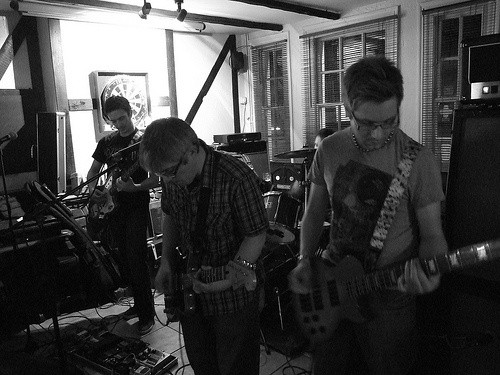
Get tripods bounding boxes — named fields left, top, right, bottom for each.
left=0, top=150, right=57, bottom=355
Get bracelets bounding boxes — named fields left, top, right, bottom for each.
left=298, top=254, right=311, bottom=263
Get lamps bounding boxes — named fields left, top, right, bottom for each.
left=175, top=0, right=187, bottom=21
left=138, top=0, right=151, bottom=19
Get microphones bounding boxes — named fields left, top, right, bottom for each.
left=0, top=131, right=18, bottom=144
left=467, top=332, right=493, bottom=344
left=110, top=142, right=141, bottom=158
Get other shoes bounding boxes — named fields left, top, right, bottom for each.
left=136, top=315, right=153, bottom=334
left=124, top=305, right=137, bottom=316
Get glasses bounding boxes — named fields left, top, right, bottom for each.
left=153, top=156, right=183, bottom=178
left=350, top=108, right=400, bottom=131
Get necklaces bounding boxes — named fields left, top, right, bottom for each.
left=352, top=131, right=394, bottom=152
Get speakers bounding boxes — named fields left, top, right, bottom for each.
left=231, top=52, right=244, bottom=69
left=443, top=104, right=500, bottom=375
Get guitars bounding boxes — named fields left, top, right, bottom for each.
left=164, top=255, right=257, bottom=323
left=88, top=157, right=144, bottom=220
left=292, top=240, right=499, bottom=345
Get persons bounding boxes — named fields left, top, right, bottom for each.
left=87, top=96, right=156, bottom=334
left=289, top=55, right=447, bottom=375
left=291, top=128, right=336, bottom=222
left=139, top=118, right=271, bottom=375
